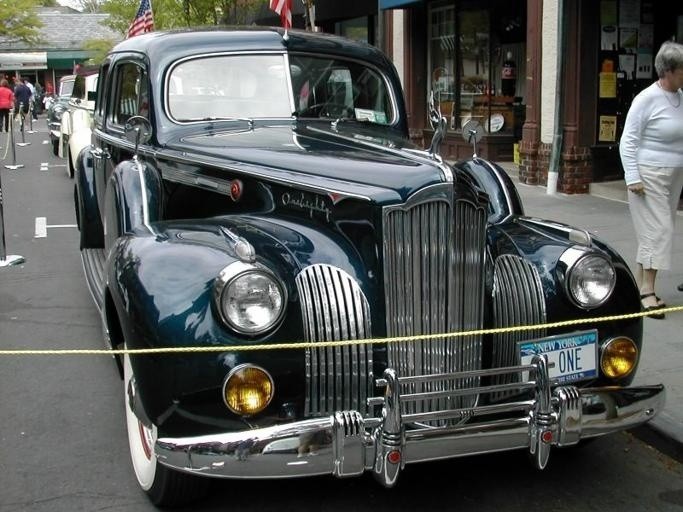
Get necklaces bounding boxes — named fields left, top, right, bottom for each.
left=658, top=80, right=681, bottom=108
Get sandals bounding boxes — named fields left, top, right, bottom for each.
left=640, top=292, right=665, bottom=319
left=657, top=297, right=667, bottom=310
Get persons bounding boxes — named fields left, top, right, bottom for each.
left=0, top=79, right=14, bottom=133
left=13, top=78, right=32, bottom=132
left=19, top=77, right=38, bottom=127
left=619, top=41, right=683, bottom=320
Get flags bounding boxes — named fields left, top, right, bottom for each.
left=126, top=0, right=155, bottom=38
left=270, top=0, right=293, bottom=27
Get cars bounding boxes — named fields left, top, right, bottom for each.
left=58, top=65, right=98, bottom=178
left=73, top=26, right=665, bottom=508
left=47, top=75, right=76, bottom=154
